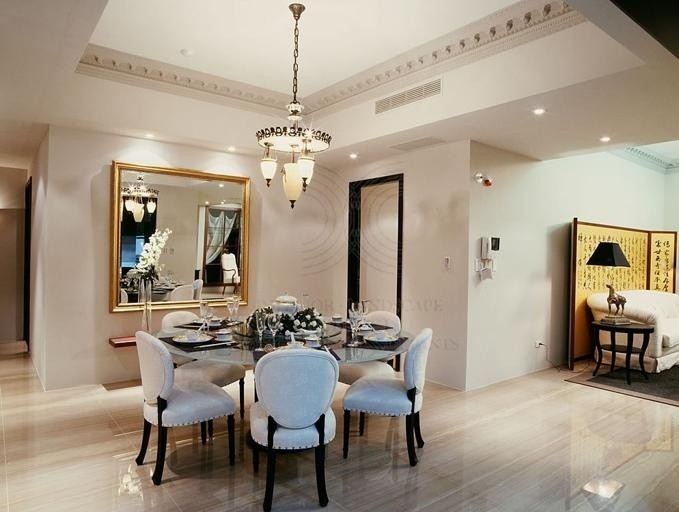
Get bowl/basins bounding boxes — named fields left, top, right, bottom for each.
left=304, top=337, right=321, bottom=348
left=331, top=315, right=343, bottom=325
left=186, top=330, right=200, bottom=341
left=374, top=330, right=386, bottom=340
left=215, top=329, right=233, bottom=341
left=211, top=318, right=222, bottom=326
left=287, top=341, right=304, bottom=348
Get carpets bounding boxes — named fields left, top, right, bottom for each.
left=562, top=363, right=679, bottom=407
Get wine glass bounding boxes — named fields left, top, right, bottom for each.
left=225, top=295, right=240, bottom=326
left=254, top=310, right=266, bottom=352
left=267, top=313, right=281, bottom=350
left=199, top=301, right=214, bottom=335
left=346, top=299, right=373, bottom=348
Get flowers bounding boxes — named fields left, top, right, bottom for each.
left=124, top=227, right=174, bottom=330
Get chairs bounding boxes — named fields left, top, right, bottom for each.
left=218, top=250, right=241, bottom=295
left=164, top=278, right=203, bottom=303
left=587, top=287, right=678, bottom=375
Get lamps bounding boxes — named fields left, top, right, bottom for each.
left=254, top=3, right=332, bottom=213
left=120, top=170, right=161, bottom=223
left=474, top=170, right=495, bottom=187
left=585, top=241, right=634, bottom=325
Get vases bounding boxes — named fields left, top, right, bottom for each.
left=139, top=276, right=153, bottom=334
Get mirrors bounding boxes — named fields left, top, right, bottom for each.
left=107, top=159, right=252, bottom=313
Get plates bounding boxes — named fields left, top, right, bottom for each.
left=363, top=335, right=399, bottom=343
left=171, top=334, right=214, bottom=344
left=192, top=317, right=223, bottom=324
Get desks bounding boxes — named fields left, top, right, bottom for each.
left=589, top=319, right=656, bottom=385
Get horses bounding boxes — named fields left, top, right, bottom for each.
left=605, top=282, right=627, bottom=317
